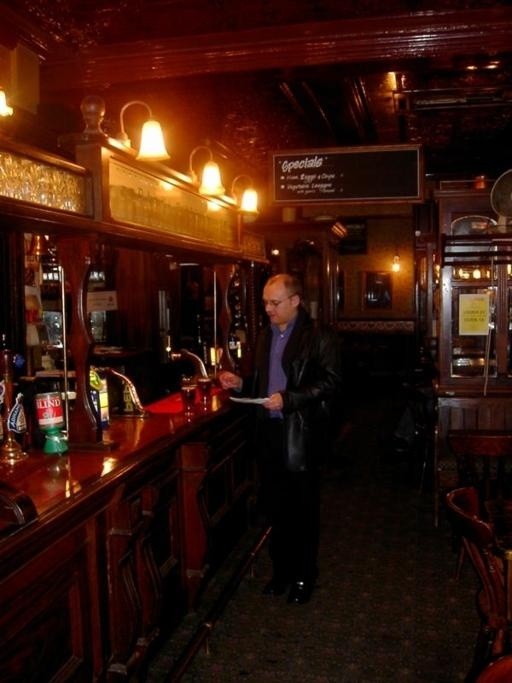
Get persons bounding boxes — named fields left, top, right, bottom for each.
left=218, top=272, right=342, bottom=606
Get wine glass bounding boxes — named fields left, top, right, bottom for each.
left=181, top=378, right=213, bottom=423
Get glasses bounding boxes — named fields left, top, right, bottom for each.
left=263, top=291, right=297, bottom=307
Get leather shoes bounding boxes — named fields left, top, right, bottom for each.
left=261, top=575, right=316, bottom=606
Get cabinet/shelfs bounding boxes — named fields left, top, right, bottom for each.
left=416, top=180, right=512, bottom=526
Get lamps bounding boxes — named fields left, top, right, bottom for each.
left=232, top=175, right=260, bottom=215
left=0, top=90, right=13, bottom=116
left=116, top=100, right=171, bottom=163
left=184, top=145, right=226, bottom=197
left=392, top=253, right=400, bottom=272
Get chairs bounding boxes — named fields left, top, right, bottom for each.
left=445, top=487, right=512, bottom=675
